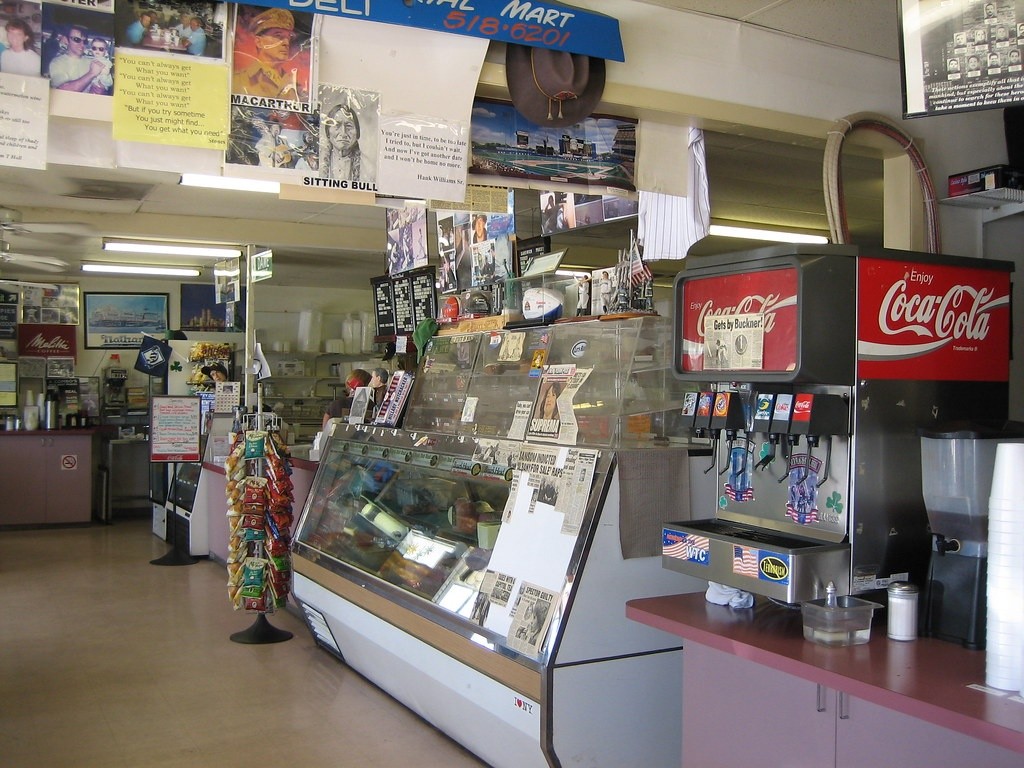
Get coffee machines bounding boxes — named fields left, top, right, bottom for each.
left=102, top=366, right=129, bottom=416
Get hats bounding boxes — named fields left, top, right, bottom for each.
left=472, top=214, right=487, bottom=230
left=201, top=363, right=227, bottom=380
left=505, top=43, right=606, bottom=128
left=412, top=316, right=438, bottom=364
left=249, top=7, right=298, bottom=35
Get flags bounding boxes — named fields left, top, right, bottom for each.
left=631, top=233, right=653, bottom=289
left=133, top=335, right=173, bottom=377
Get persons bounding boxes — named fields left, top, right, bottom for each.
left=201, top=363, right=227, bottom=392
left=599, top=271, right=612, bottom=315
left=0, top=18, right=41, bottom=77
left=948, top=3, right=1024, bottom=74
left=529, top=382, right=560, bottom=435
left=44, top=25, right=114, bottom=94
left=573, top=273, right=589, bottom=317
left=255, top=120, right=296, bottom=168
left=544, top=195, right=560, bottom=234
left=126, top=10, right=206, bottom=56
left=318, top=106, right=374, bottom=183
left=442, top=254, right=456, bottom=291
left=233, top=8, right=302, bottom=101
left=372, top=368, right=388, bottom=410
left=322, top=369, right=376, bottom=431
left=472, top=214, right=487, bottom=243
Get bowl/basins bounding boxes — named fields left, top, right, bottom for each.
left=800, top=595, right=884, bottom=648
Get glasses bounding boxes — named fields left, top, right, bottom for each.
left=91, top=46, right=104, bottom=51
left=69, top=36, right=88, bottom=45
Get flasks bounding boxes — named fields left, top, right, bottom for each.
left=44, top=389, right=57, bottom=430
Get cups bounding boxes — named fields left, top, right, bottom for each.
left=477, top=521, right=501, bottom=549
left=293, top=422, right=300, bottom=439
left=26, top=390, right=33, bottom=405
left=986, top=443, right=1024, bottom=692
left=282, top=429, right=288, bottom=446
left=37, top=394, right=44, bottom=422
left=288, top=433, right=295, bottom=444
left=331, top=364, right=338, bottom=377
left=124, top=436, right=129, bottom=440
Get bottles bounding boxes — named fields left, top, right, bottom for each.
left=6, top=416, right=14, bottom=431
left=24, top=412, right=39, bottom=432
left=232, top=410, right=244, bottom=448
left=58, top=413, right=62, bottom=430
left=111, top=354, right=120, bottom=369
left=886, top=581, right=920, bottom=641
left=80, top=410, right=88, bottom=429
left=15, top=416, right=22, bottom=430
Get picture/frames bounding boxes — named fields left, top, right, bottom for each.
left=83, top=291, right=169, bottom=349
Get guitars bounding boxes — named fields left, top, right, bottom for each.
left=258, top=144, right=306, bottom=169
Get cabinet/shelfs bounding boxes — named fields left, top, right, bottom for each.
left=0, top=434, right=95, bottom=532
left=682, top=637, right=1024, bottom=768
